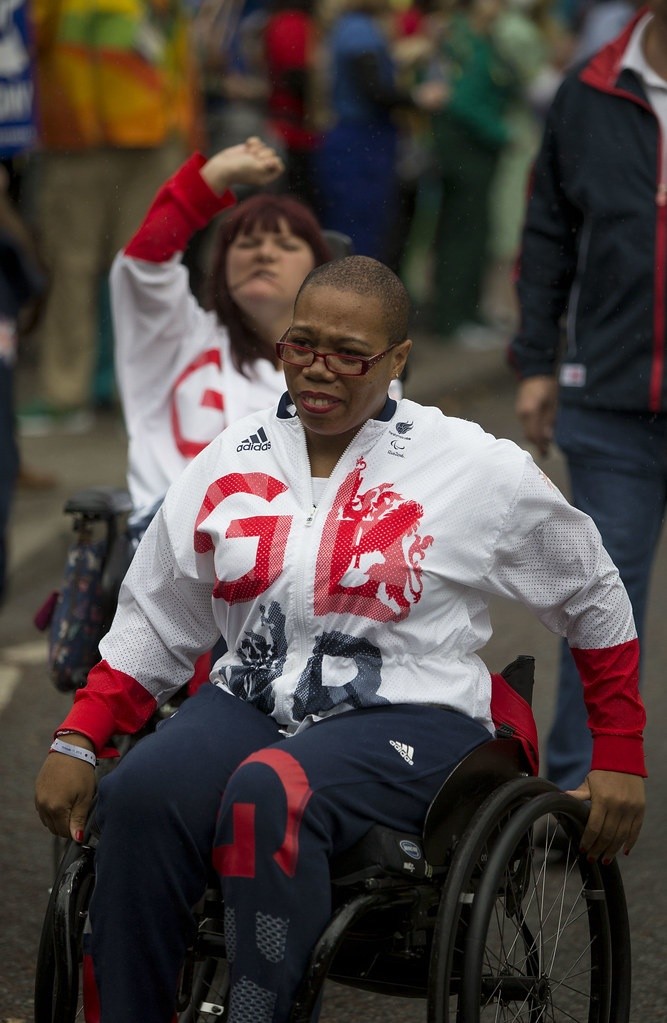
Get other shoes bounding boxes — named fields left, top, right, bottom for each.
left=534, top=812, right=577, bottom=850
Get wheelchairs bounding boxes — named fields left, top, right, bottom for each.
left=32, top=654, right=632, bottom=1023
left=47, top=488, right=136, bottom=692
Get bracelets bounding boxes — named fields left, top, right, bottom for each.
left=49, top=738, right=96, bottom=767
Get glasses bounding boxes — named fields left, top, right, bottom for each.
left=276, top=325, right=401, bottom=376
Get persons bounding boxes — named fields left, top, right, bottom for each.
left=1, top=0, right=638, bottom=609
left=109, top=136, right=329, bottom=715
left=504, top=0, right=667, bottom=867
left=32, top=255, right=648, bottom=1023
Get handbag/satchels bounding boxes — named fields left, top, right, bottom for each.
left=46, top=537, right=128, bottom=693
